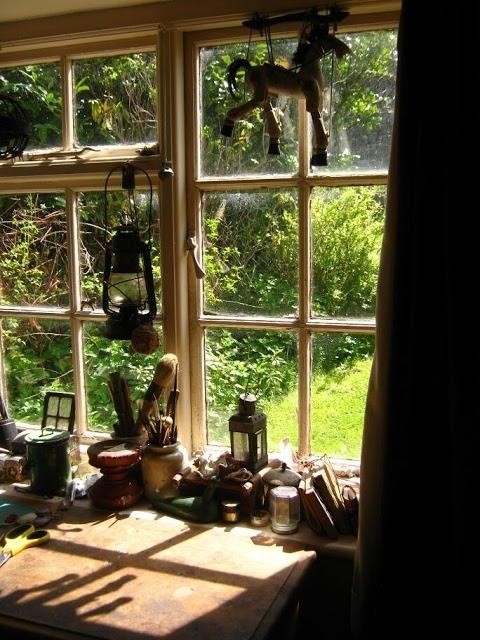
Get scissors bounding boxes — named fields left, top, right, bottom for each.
left=0, top=523, right=50, bottom=568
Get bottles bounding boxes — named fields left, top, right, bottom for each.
left=221, top=499, right=240, bottom=524
left=269, top=486, right=301, bottom=536
left=24, top=428, right=71, bottom=492
left=142, top=441, right=182, bottom=499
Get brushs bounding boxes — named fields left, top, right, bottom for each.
left=130, top=352, right=178, bottom=437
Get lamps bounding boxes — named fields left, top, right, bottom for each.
left=103, top=163, right=156, bottom=340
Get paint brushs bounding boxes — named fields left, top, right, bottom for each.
left=136, top=389, right=180, bottom=448
left=107, top=372, right=135, bottom=437
